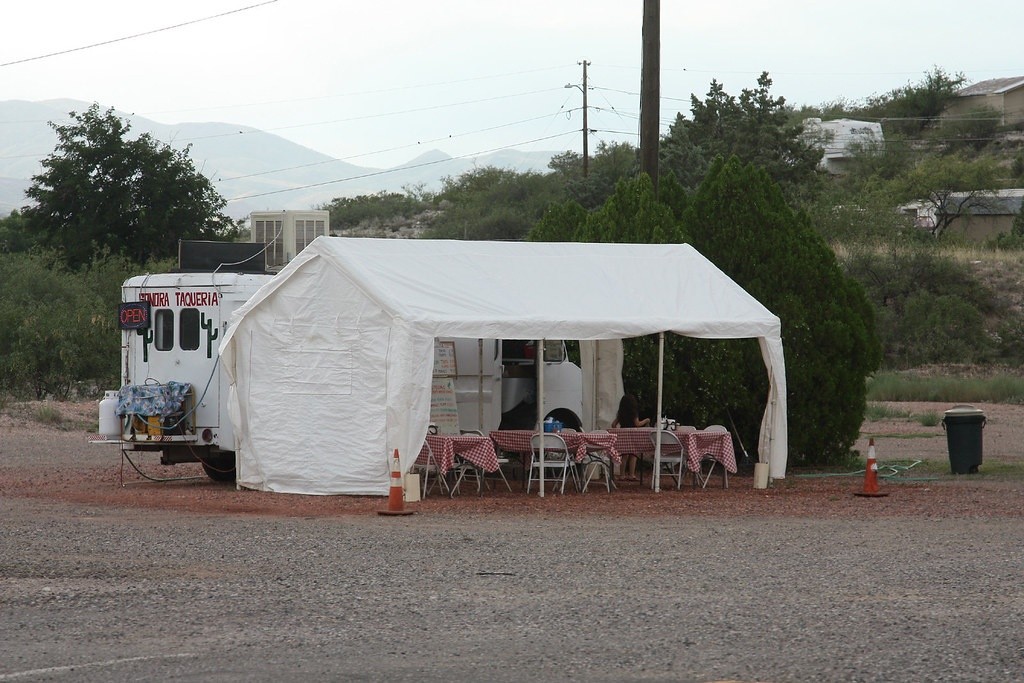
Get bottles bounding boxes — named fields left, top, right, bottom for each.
left=553, top=424, right=560, bottom=433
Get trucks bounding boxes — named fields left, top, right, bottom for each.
left=89, top=208, right=582, bottom=481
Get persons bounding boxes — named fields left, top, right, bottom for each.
left=612, top=394, right=650, bottom=481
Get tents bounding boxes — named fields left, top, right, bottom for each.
left=216, top=235, right=788, bottom=497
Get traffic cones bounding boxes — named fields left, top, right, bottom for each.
left=377, top=448, right=414, bottom=517
left=853, top=438, right=890, bottom=497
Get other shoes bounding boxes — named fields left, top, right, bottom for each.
left=629, top=477, right=641, bottom=482
left=619, top=477, right=629, bottom=481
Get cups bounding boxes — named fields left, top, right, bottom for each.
left=661, top=419, right=677, bottom=431
